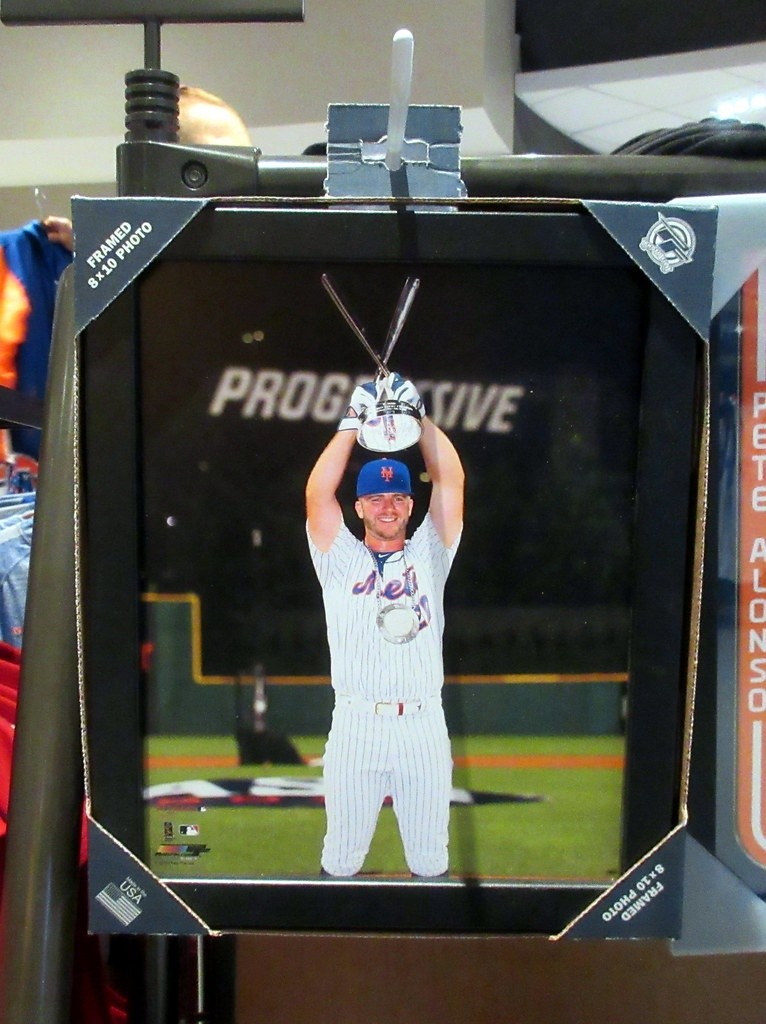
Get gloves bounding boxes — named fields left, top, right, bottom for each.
left=336, top=375, right=384, bottom=430
left=384, top=373, right=426, bottom=419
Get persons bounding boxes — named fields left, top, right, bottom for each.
left=305, top=376, right=466, bottom=880
left=45, top=214, right=73, bottom=255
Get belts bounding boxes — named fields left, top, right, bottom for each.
left=338, top=695, right=442, bottom=717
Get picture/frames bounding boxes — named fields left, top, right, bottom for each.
left=69, top=198, right=717, bottom=940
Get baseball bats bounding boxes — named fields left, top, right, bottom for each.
left=372, top=274, right=423, bottom=384
left=319, top=271, right=391, bottom=377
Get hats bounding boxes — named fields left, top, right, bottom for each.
left=354, top=457, right=415, bottom=498
